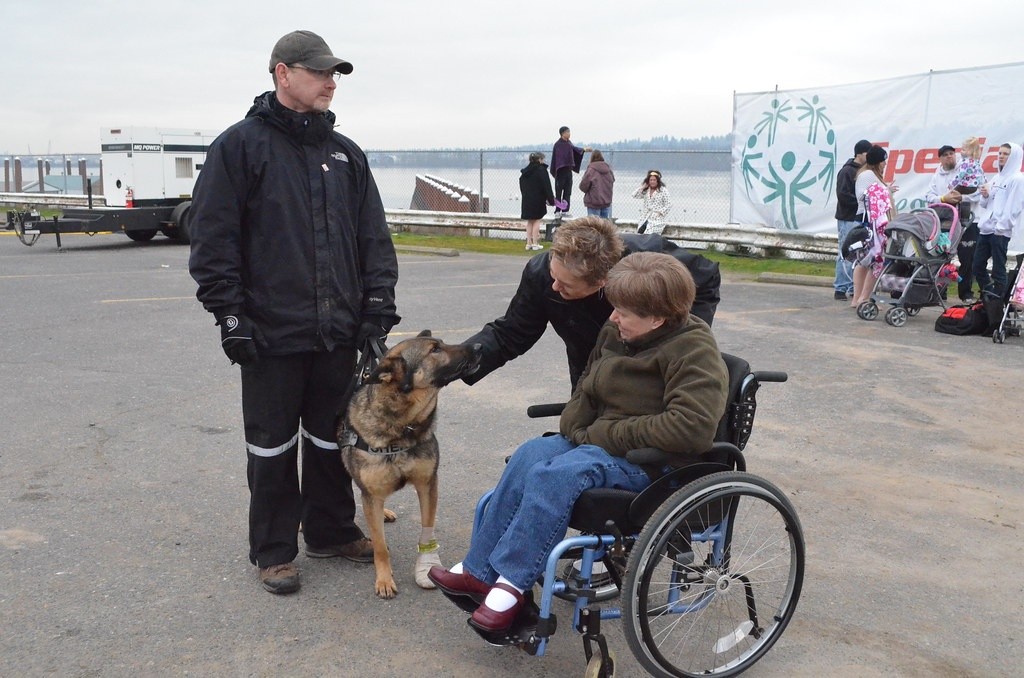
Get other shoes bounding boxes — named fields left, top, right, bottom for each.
left=561, top=211, right=573, bottom=218
left=525, top=244, right=532, bottom=250
left=532, top=243, right=543, bottom=251
left=834, top=290, right=848, bottom=301
left=963, top=299, right=973, bottom=306
left=555, top=211, right=560, bottom=218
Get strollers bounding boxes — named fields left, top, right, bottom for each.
left=992, top=254, right=1024, bottom=344
left=856, top=201, right=976, bottom=328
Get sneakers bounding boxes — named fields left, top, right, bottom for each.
left=304, top=537, right=374, bottom=562
left=256, top=562, right=299, bottom=593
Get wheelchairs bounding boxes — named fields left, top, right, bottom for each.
left=441, top=350, right=806, bottom=678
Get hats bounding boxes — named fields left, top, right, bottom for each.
left=938, top=146, right=955, bottom=157
left=866, top=145, right=888, bottom=164
left=559, top=127, right=569, bottom=134
left=268, top=29, right=353, bottom=75
left=854, top=140, right=872, bottom=154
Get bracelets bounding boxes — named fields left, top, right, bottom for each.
left=941, top=195, right=945, bottom=202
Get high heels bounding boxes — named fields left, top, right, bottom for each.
left=427, top=565, right=492, bottom=605
left=469, top=582, right=527, bottom=634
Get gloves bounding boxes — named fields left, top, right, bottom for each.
left=220, top=305, right=270, bottom=366
left=355, top=322, right=387, bottom=358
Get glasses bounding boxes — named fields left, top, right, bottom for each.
left=285, top=65, right=341, bottom=83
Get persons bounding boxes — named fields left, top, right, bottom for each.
left=851, top=145, right=899, bottom=311
left=189, top=30, right=401, bottom=594
left=631, top=170, right=672, bottom=236
left=927, top=137, right=988, bottom=303
left=579, top=149, right=615, bottom=218
left=550, top=126, right=592, bottom=219
left=833, top=139, right=872, bottom=300
left=459, top=214, right=721, bottom=580
left=428, top=252, right=729, bottom=628
left=971, top=142, right=1024, bottom=300
left=519, top=152, right=554, bottom=251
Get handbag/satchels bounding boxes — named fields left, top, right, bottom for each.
left=546, top=219, right=561, bottom=241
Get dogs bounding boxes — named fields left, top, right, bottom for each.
left=335, top=328, right=484, bottom=602
